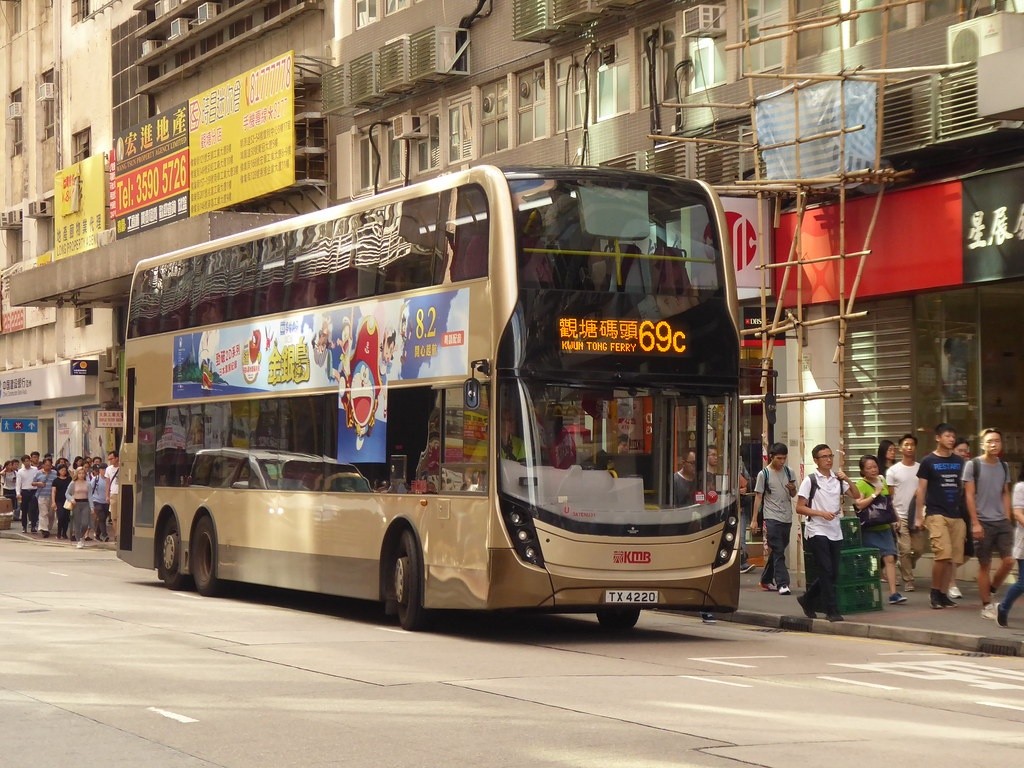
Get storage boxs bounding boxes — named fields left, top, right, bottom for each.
left=801, top=517, right=883, bottom=615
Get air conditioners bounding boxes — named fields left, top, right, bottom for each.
left=28, top=201, right=52, bottom=216
left=417, top=30, right=456, bottom=74
left=171, top=18, right=189, bottom=37
left=384, top=33, right=421, bottom=85
left=683, top=4, right=727, bottom=38
left=520, top=0, right=642, bottom=40
left=393, top=116, right=429, bottom=139
left=142, top=40, right=157, bottom=56
left=354, top=68, right=381, bottom=97
left=0, top=209, right=21, bottom=229
left=947, top=11, right=1024, bottom=65
left=39, top=83, right=54, bottom=99
left=9, top=102, right=21, bottom=118
left=198, top=2, right=217, bottom=25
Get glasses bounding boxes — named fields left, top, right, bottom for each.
left=708, top=453, right=718, bottom=458
left=817, top=454, right=835, bottom=460
left=684, top=460, right=696, bottom=468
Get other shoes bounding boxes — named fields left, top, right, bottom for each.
left=700, top=612, right=716, bottom=623
left=896, top=580, right=901, bottom=586
left=797, top=596, right=818, bottom=618
left=881, top=576, right=887, bottom=582
left=939, top=594, right=958, bottom=607
left=824, top=609, right=843, bottom=621
left=22, top=526, right=110, bottom=549
left=930, top=598, right=944, bottom=608
left=904, top=580, right=915, bottom=592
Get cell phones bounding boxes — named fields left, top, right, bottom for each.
left=789, top=480, right=796, bottom=484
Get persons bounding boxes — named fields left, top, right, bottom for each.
left=795, top=444, right=860, bottom=621
left=915, top=423, right=971, bottom=609
left=0, top=451, right=119, bottom=548
left=672, top=445, right=756, bottom=574
left=750, top=443, right=798, bottom=595
left=878, top=434, right=926, bottom=592
left=854, top=455, right=906, bottom=604
left=961, top=428, right=1016, bottom=621
left=618, top=434, right=631, bottom=455
left=466, top=409, right=527, bottom=492
left=545, top=192, right=594, bottom=292
left=996, top=464, right=1024, bottom=627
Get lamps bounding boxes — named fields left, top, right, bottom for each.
left=55, top=295, right=65, bottom=309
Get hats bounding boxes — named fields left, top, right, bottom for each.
left=93, top=464, right=100, bottom=470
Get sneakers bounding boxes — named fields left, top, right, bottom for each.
left=981, top=604, right=995, bottom=619
left=778, top=585, right=791, bottom=594
left=994, top=602, right=1008, bottom=627
left=948, top=586, right=962, bottom=598
left=888, top=593, right=907, bottom=603
left=759, top=580, right=778, bottom=591
left=740, top=563, right=756, bottom=573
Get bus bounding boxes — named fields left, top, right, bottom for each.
left=116, top=162, right=781, bottom=633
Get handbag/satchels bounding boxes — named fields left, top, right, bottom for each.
left=63, top=498, right=73, bottom=511
left=853, top=480, right=897, bottom=528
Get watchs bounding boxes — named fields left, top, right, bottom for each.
left=871, top=494, right=877, bottom=498
